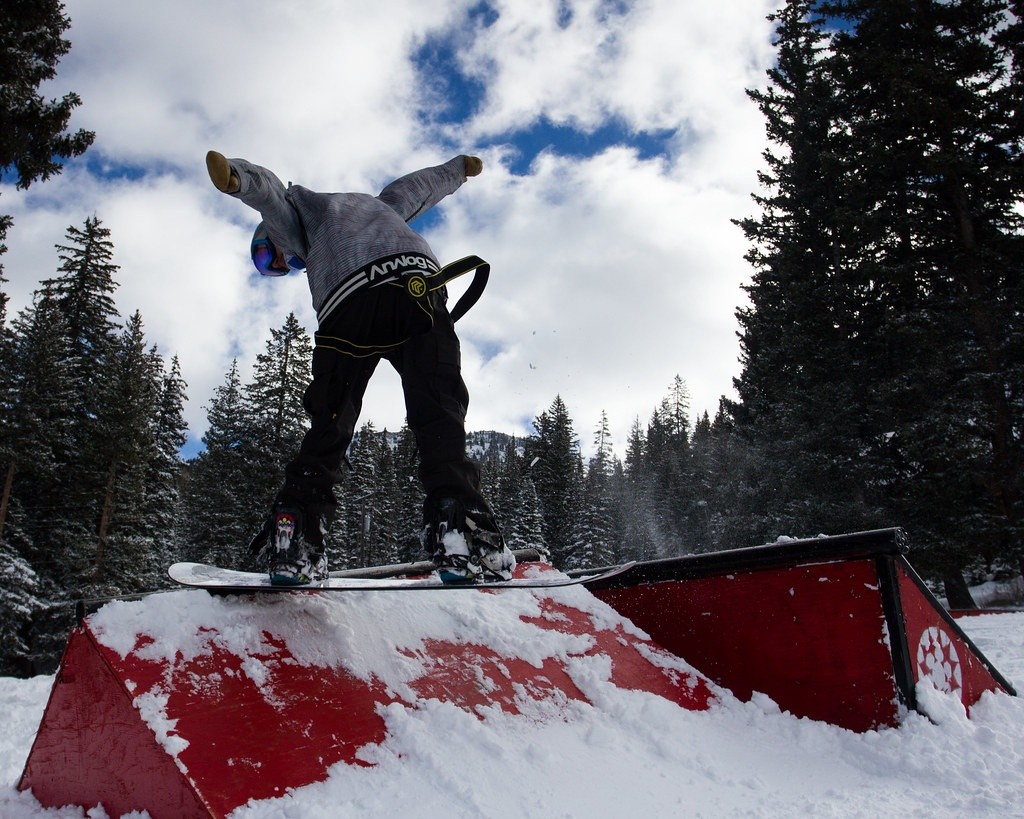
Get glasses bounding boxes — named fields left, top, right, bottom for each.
left=252, top=240, right=287, bottom=276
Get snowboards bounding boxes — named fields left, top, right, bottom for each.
left=167, top=560, right=637, bottom=590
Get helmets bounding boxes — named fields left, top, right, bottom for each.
left=251, top=219, right=301, bottom=278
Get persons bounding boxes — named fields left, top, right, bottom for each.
left=206, top=149, right=515, bottom=586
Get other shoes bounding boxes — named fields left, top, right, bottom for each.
left=439, top=551, right=516, bottom=583
left=270, top=515, right=328, bottom=584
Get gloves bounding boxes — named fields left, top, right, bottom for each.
left=462, top=154, right=483, bottom=177
left=206, top=150, right=240, bottom=193
